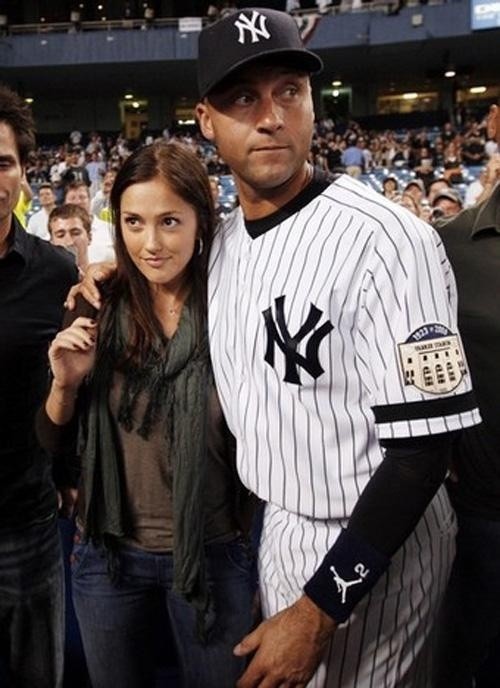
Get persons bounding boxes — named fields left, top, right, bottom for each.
left=144, top=7, right=154, bottom=28
left=14, top=131, right=116, bottom=273
left=316, top=1, right=443, bottom=13
left=58, top=6, right=484, bottom=687
left=110, top=130, right=184, bottom=169
left=431, top=105, right=500, bottom=688
left=183, top=135, right=239, bottom=219
left=209, top=6, right=236, bottom=21
left=286, top=1, right=299, bottom=13
left=29, top=142, right=273, bottom=686
left=308, top=107, right=498, bottom=226
left=71, top=11, right=81, bottom=33
left=1, top=87, right=86, bottom=686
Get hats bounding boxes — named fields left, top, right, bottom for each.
left=431, top=189, right=462, bottom=206
left=197, top=8, right=323, bottom=103
left=68, top=146, right=81, bottom=154
left=61, top=167, right=90, bottom=187
